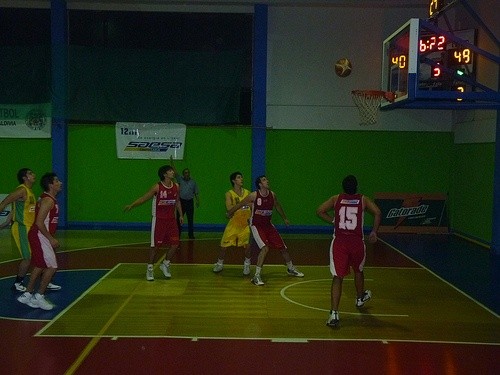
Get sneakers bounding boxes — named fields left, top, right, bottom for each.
left=326, top=309, right=340, bottom=327
left=146, top=265, right=155, bottom=281
left=355, top=289, right=372, bottom=308
left=286, top=265, right=305, bottom=278
left=159, top=260, right=172, bottom=278
left=29, top=293, right=55, bottom=310
left=13, top=280, right=28, bottom=292
left=17, top=291, right=40, bottom=309
left=251, top=273, right=264, bottom=286
left=45, top=283, right=62, bottom=291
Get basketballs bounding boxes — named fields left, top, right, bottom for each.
left=335, top=57, right=352, bottom=77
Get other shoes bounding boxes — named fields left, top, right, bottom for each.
left=188, top=235, right=195, bottom=239
left=242, top=260, right=251, bottom=275
left=212, top=262, right=224, bottom=272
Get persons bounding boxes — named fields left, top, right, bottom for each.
left=317, top=176, right=381, bottom=327
left=17, top=173, right=62, bottom=310
left=213, top=172, right=252, bottom=276
left=228, top=176, right=305, bottom=285
left=176, top=169, right=200, bottom=239
left=124, top=166, right=184, bottom=281
left=0, top=168, right=62, bottom=292
